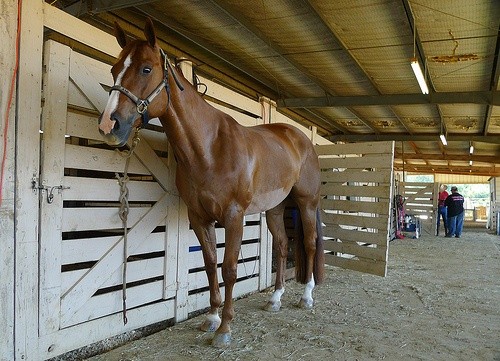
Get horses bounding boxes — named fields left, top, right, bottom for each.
left=96, top=17, right=325, bottom=348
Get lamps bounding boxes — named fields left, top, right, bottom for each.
left=409, top=17, right=430, bottom=95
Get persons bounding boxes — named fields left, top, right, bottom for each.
left=432, top=184, right=465, bottom=239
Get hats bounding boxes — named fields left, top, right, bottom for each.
left=450, top=186, right=458, bottom=191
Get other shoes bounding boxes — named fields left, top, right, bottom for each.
left=455, top=235, right=461, bottom=238
left=445, top=235, right=455, bottom=238
